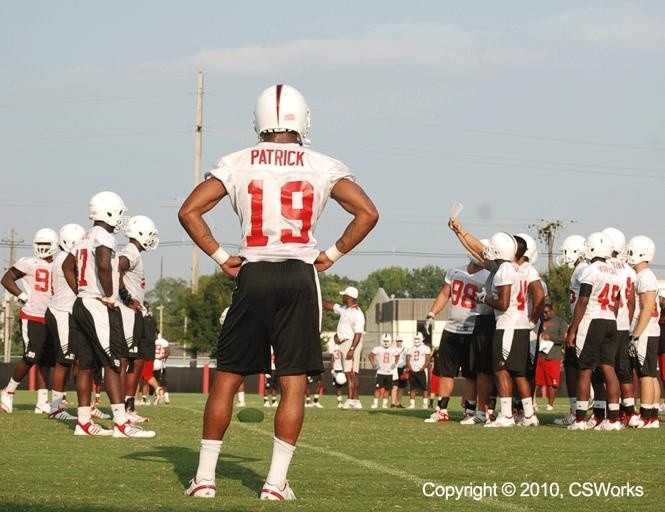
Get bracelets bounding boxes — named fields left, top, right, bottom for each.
left=209, top=248, right=230, bottom=265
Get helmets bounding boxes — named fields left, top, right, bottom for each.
left=412, top=331, right=424, bottom=346
left=126, top=213, right=159, bottom=251
left=253, top=84, right=311, bottom=144
left=467, top=231, right=538, bottom=266
left=60, top=223, right=86, bottom=252
left=556, top=227, right=657, bottom=267
left=380, top=333, right=392, bottom=348
left=32, top=227, right=59, bottom=259
left=88, top=191, right=129, bottom=233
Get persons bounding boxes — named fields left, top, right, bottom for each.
left=218, top=219, right=664, bottom=430
left=3, top=186, right=169, bottom=438
left=178, top=82, right=379, bottom=501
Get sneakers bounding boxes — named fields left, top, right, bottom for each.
left=554, top=413, right=661, bottom=431
left=235, top=400, right=245, bottom=407
left=460, top=403, right=539, bottom=429
left=546, top=404, right=553, bottom=411
left=423, top=408, right=449, bottom=423
left=34, top=386, right=170, bottom=439
left=339, top=402, right=439, bottom=412
left=183, top=476, right=218, bottom=498
left=260, top=480, right=297, bottom=500
left=264, top=400, right=323, bottom=408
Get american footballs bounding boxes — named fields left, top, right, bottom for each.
left=237, top=409, right=264, bottom=423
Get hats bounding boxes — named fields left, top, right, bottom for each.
left=338, top=287, right=359, bottom=298
